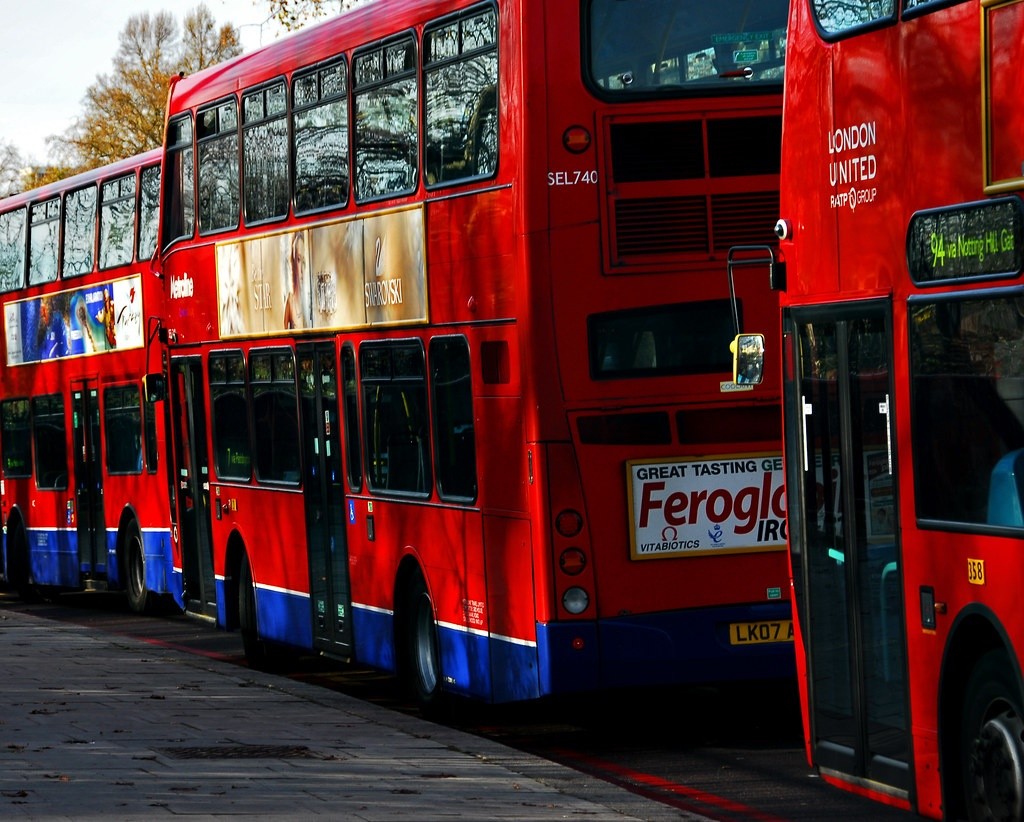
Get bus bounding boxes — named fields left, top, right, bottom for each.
left=142, top=2, right=786, bottom=725
left=0, top=145, right=176, bottom=613
left=727, top=1, right=1021, bottom=822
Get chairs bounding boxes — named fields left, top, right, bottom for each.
left=986, top=450, right=1024, bottom=528
